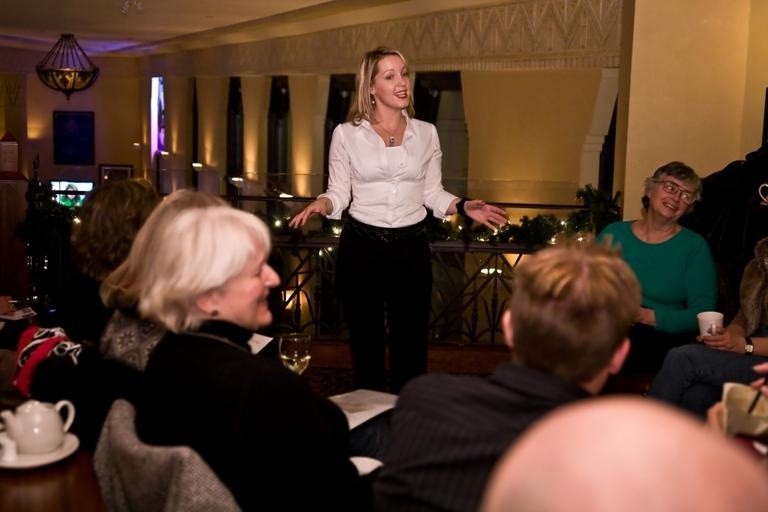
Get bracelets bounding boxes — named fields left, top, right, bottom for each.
left=455, top=198, right=471, bottom=215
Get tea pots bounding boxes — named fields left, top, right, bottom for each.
left=1, top=400, right=76, bottom=455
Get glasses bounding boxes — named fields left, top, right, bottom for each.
left=654, top=178, right=698, bottom=206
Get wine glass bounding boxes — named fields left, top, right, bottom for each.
left=280, top=334, right=311, bottom=375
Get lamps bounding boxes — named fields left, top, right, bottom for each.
left=36, top=33, right=99, bottom=101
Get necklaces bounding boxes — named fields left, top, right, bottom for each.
left=376, top=118, right=401, bottom=147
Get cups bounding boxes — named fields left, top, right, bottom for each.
left=697, top=311, right=724, bottom=338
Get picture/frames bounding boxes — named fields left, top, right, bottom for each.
left=99, top=164, right=133, bottom=189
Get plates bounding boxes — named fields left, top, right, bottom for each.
left=0, top=431, right=80, bottom=470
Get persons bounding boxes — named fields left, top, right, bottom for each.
left=140, top=205, right=365, bottom=512
left=593, top=161, right=717, bottom=395
left=37, top=178, right=160, bottom=348
left=705, top=362, right=768, bottom=456
left=368, top=243, right=642, bottom=511
left=647, top=183, right=767, bottom=420
left=480, top=396, right=768, bottom=512
left=97, top=188, right=233, bottom=371
left=289, top=45, right=509, bottom=398
left=61, top=184, right=83, bottom=207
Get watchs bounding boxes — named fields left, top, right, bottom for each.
left=744, top=336, right=754, bottom=356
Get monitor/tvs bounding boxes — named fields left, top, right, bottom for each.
left=47, top=179, right=96, bottom=208
